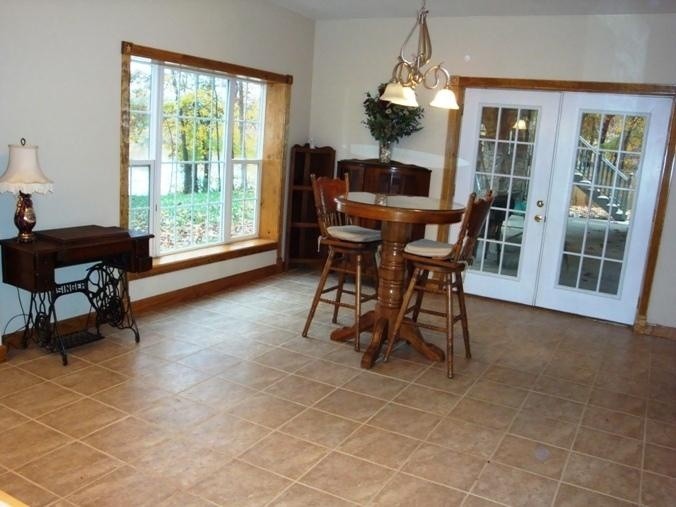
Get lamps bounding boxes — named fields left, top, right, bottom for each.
left=379, top=0, right=460, bottom=109
left=0, top=138, right=54, bottom=243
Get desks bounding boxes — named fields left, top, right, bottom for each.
left=334, top=190, right=466, bottom=369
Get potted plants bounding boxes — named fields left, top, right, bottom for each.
left=360, top=84, right=424, bottom=163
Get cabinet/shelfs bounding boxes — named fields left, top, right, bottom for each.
left=285, top=143, right=335, bottom=272
left=337, top=158, right=432, bottom=242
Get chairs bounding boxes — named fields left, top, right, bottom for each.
left=302, top=173, right=379, bottom=353
left=384, top=191, right=494, bottom=379
left=473, top=194, right=516, bottom=265
